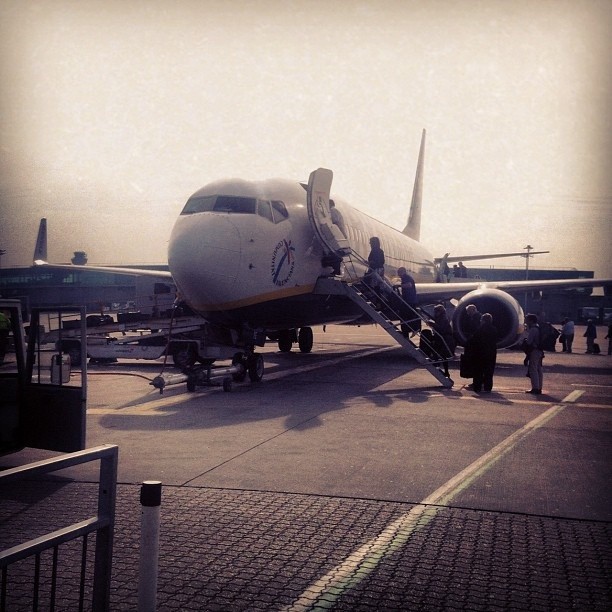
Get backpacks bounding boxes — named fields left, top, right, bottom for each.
left=531, top=322, right=560, bottom=352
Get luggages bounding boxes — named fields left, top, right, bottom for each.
left=399, top=307, right=422, bottom=332
left=420, top=329, right=432, bottom=356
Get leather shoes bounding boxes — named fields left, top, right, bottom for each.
left=537, top=389, right=543, bottom=394
left=525, top=389, right=539, bottom=394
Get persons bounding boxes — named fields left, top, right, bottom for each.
left=537, top=318, right=612, bottom=355
left=362, top=236, right=385, bottom=306
left=522, top=312, right=546, bottom=395
left=464, top=303, right=497, bottom=392
left=427, top=305, right=454, bottom=378
left=441, top=262, right=471, bottom=278
left=323, top=197, right=345, bottom=280
left=390, top=266, right=420, bottom=341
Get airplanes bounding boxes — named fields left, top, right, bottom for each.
left=0, top=218, right=180, bottom=318
left=28, top=128, right=612, bottom=392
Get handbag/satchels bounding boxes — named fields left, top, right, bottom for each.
left=439, top=337, right=457, bottom=357
left=559, top=335, right=563, bottom=343
left=388, top=285, right=401, bottom=310
left=459, top=354, right=485, bottom=379
left=593, top=343, right=600, bottom=353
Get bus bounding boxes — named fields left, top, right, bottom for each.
left=580, top=307, right=612, bottom=324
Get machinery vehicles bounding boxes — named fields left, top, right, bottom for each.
left=0, top=296, right=90, bottom=457
